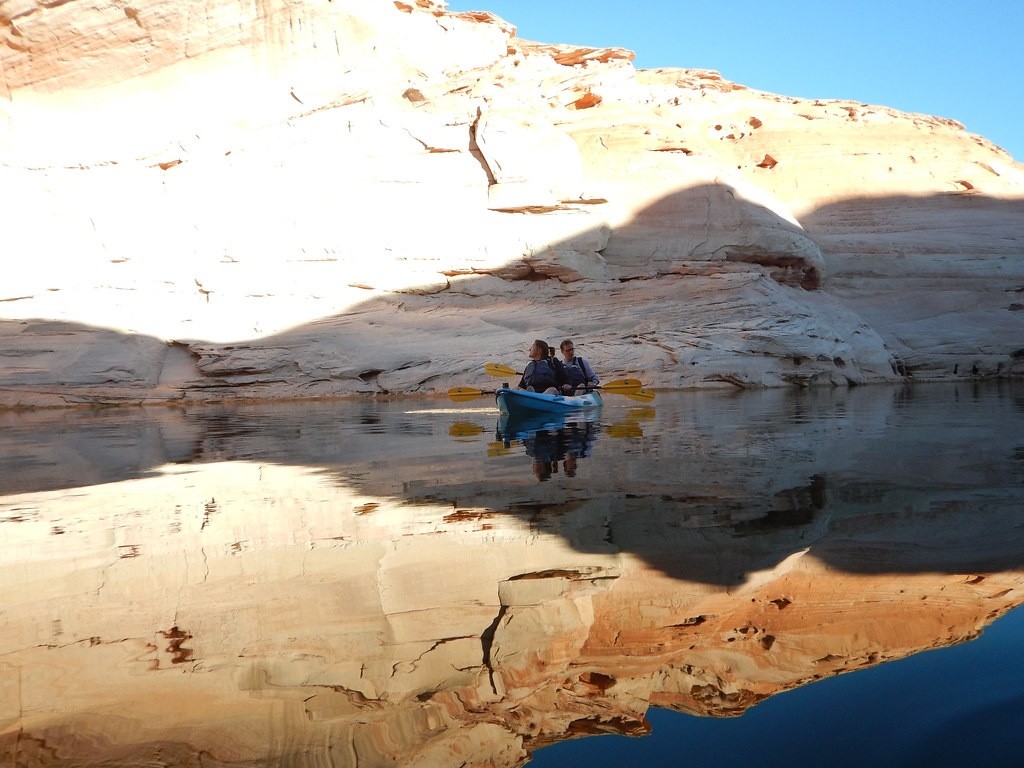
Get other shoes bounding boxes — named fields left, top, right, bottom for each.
left=526, top=386, right=535, bottom=392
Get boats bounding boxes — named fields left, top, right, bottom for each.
left=493, top=407, right=603, bottom=448
left=493, top=382, right=605, bottom=414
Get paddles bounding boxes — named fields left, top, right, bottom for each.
left=447, top=361, right=657, bottom=402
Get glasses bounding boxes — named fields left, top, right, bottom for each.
left=564, top=348, right=574, bottom=353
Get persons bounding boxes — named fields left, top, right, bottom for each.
left=514, top=340, right=572, bottom=395
left=559, top=338, right=599, bottom=395
